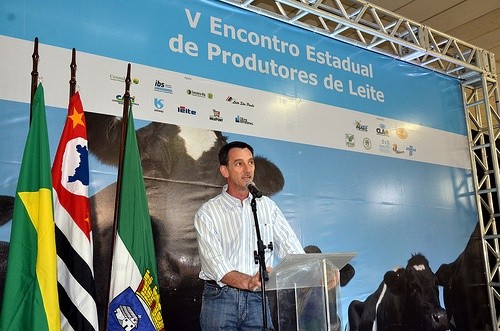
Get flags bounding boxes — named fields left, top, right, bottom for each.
left=106, top=96, right=166, bottom=331
left=0, top=76, right=61, bottom=331
left=51, top=91, right=99, bottom=331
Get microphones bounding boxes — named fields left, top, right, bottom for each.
left=246, top=182, right=262, bottom=198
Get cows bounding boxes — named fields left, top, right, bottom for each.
left=348, top=253, right=455, bottom=331
left=71, top=112, right=356, bottom=331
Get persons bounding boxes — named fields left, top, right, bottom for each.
left=193, top=141, right=340, bottom=331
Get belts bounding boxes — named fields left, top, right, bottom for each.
left=208, top=279, right=237, bottom=289
